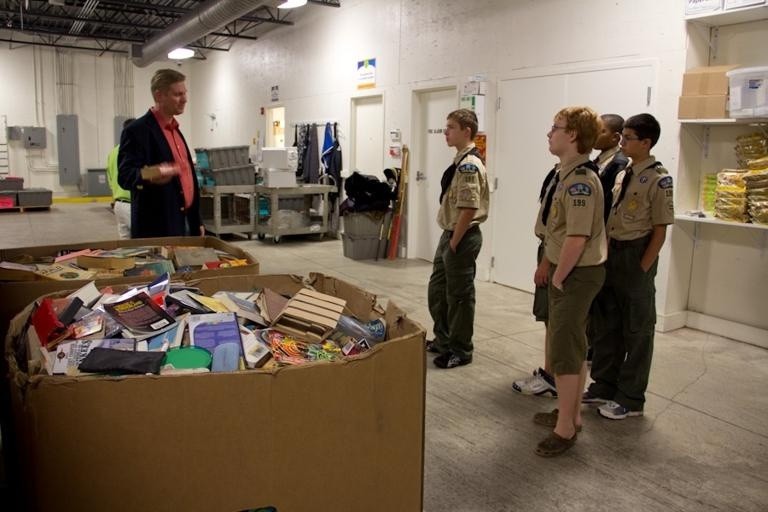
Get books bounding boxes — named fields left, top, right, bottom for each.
left=0, top=246, right=348, bottom=372
left=139, top=162, right=188, bottom=179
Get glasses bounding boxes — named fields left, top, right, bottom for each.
left=552, top=124, right=566, bottom=131
left=621, top=135, right=639, bottom=142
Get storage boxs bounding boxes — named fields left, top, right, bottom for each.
left=677, top=95, right=728, bottom=119
left=339, top=232, right=388, bottom=260
left=0, top=178, right=53, bottom=208
left=725, top=66, right=768, bottom=119
left=0, top=235, right=261, bottom=355
left=724, top=0, right=766, bottom=10
left=681, top=64, right=741, bottom=94
left=685, top=0, right=724, bottom=14
left=3, top=270, right=428, bottom=512
left=342, top=210, right=384, bottom=238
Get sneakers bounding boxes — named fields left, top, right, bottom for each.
left=426, top=338, right=472, bottom=368
left=533, top=409, right=581, bottom=457
left=512, top=367, right=561, bottom=397
left=582, top=391, right=643, bottom=420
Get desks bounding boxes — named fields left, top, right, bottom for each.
left=255, top=183, right=338, bottom=245
left=198, top=184, right=257, bottom=240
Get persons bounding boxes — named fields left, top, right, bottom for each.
left=115, top=68, right=206, bottom=236
left=105, top=116, right=138, bottom=241
left=510, top=160, right=559, bottom=399
left=424, top=108, right=490, bottom=370
left=532, top=106, right=607, bottom=459
left=580, top=112, right=674, bottom=421
left=584, top=114, right=628, bottom=363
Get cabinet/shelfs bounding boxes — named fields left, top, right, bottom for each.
left=674, top=4, right=767, bottom=230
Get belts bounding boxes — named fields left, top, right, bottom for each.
left=115, top=199, right=131, bottom=202
left=609, top=239, right=651, bottom=248
left=443, top=225, right=479, bottom=237
